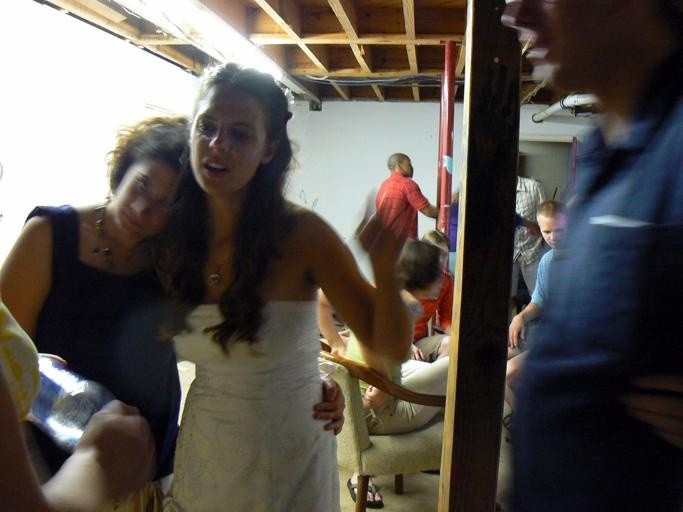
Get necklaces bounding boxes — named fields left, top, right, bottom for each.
left=206, top=238, right=228, bottom=286
left=91, top=196, right=147, bottom=272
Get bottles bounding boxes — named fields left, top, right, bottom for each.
left=23, top=351, right=114, bottom=456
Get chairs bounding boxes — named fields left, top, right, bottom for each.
left=319, top=349, right=446, bottom=512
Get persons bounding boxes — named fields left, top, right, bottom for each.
left=1, top=301, right=161, bottom=512
left=502, top=3, right=681, bottom=511
left=1, top=114, right=347, bottom=496
left=319, top=151, right=464, bottom=511
left=625, top=374, right=683, bottom=450
left=159, top=60, right=413, bottom=512
left=500, top=200, right=575, bottom=450
left=508, top=164, right=550, bottom=311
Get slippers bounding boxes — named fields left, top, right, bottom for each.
left=347, top=478, right=384, bottom=508
